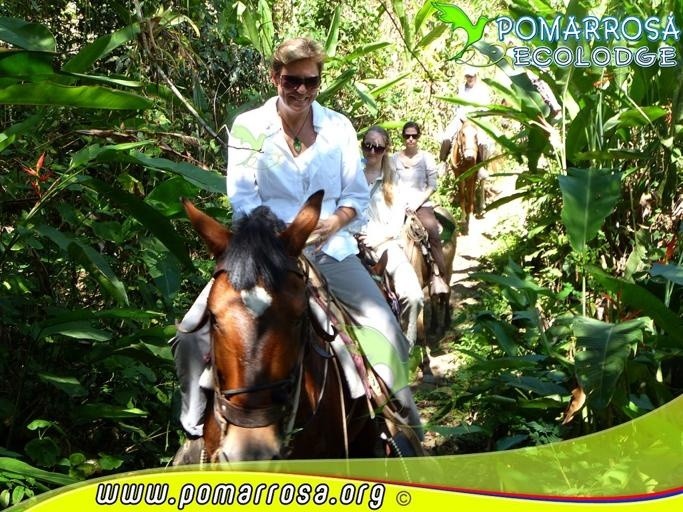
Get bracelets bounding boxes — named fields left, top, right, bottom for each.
left=337, top=208, right=350, bottom=221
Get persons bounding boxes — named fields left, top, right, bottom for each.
left=436, top=64, right=494, bottom=180
left=167, top=36, right=426, bottom=468
left=392, top=122, right=446, bottom=281
left=359, top=124, right=424, bottom=379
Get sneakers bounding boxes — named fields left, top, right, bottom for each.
left=430, top=274, right=450, bottom=299
left=171, top=430, right=204, bottom=468
left=406, top=365, right=425, bottom=387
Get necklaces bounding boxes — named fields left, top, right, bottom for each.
left=277, top=105, right=313, bottom=154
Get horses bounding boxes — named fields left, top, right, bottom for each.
left=179, top=188, right=401, bottom=473
left=449, top=117, right=487, bottom=235
left=362, top=247, right=437, bottom=386
left=400, top=207, right=458, bottom=333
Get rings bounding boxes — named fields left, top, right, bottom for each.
left=318, top=236, right=323, bottom=242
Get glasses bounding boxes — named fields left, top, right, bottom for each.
left=402, top=133, right=419, bottom=140
left=276, top=71, right=322, bottom=90
left=360, top=141, right=386, bottom=155
